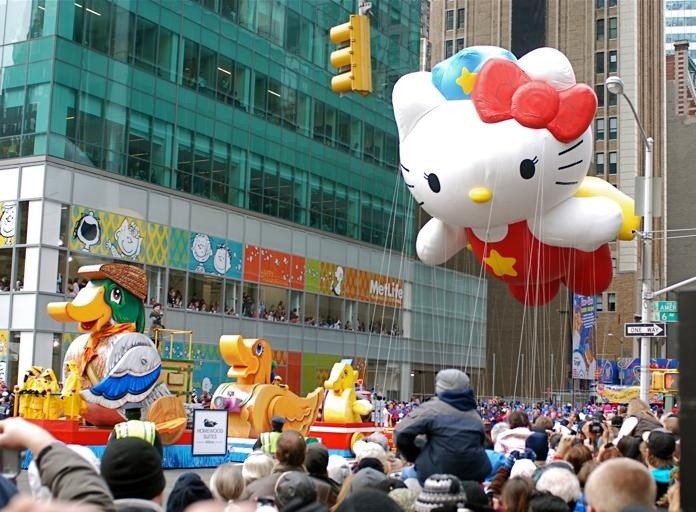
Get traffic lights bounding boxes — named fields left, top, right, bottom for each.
left=330, top=0, right=373, bottom=96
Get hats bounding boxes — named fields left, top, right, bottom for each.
left=368, top=432, right=389, bottom=449
left=434, top=368, right=471, bottom=397
left=122, top=402, right=144, bottom=412
left=531, top=460, right=581, bottom=502
left=387, top=488, right=418, bottom=512
left=352, top=439, right=388, bottom=468
left=274, top=469, right=320, bottom=512
left=272, top=417, right=286, bottom=426
left=641, top=428, right=676, bottom=457
left=509, top=456, right=536, bottom=478
left=349, top=467, right=393, bottom=501
left=99, top=437, right=167, bottom=500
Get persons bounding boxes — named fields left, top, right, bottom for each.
left=646, top=428, right=681, bottom=512
left=0, top=385, right=15, bottom=416
left=318, top=314, right=342, bottom=330
left=242, top=291, right=257, bottom=318
left=0, top=275, right=10, bottom=291
left=290, top=309, right=299, bottom=320
left=487, top=448, right=538, bottom=500
left=476, top=395, right=624, bottom=451
left=582, top=456, right=667, bottom=512
left=275, top=470, right=329, bottom=512
left=253, top=415, right=285, bottom=454
left=304, top=442, right=340, bottom=494
left=503, top=474, right=535, bottom=511
left=304, top=315, right=316, bottom=326
left=187, top=297, right=220, bottom=314
left=167, top=286, right=183, bottom=308
left=664, top=413, right=681, bottom=436
left=612, top=399, right=663, bottom=449
left=271, top=376, right=288, bottom=388
left=101, top=437, right=166, bottom=512
left=165, top=472, right=215, bottom=512
left=191, top=389, right=211, bottom=409
left=107, top=403, right=165, bottom=456
left=259, top=300, right=287, bottom=322
left=392, top=367, right=494, bottom=488
left=211, top=463, right=257, bottom=512
left=370, top=386, right=431, bottom=428
left=150, top=296, right=158, bottom=304
left=241, top=452, right=272, bottom=483
left=147, top=301, right=165, bottom=346
left=56, top=271, right=87, bottom=296
left=0, top=414, right=116, bottom=512
left=344, top=317, right=367, bottom=333
left=369, top=317, right=403, bottom=337
left=225, top=304, right=234, bottom=315
left=239, top=430, right=306, bottom=503
left=530, top=460, right=581, bottom=512
left=333, top=432, right=414, bottom=512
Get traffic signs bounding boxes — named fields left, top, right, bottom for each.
left=650, top=299, right=680, bottom=323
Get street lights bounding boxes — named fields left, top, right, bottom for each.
left=606, top=75, right=696, bottom=402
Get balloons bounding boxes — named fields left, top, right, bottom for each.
left=388, top=44, right=641, bottom=307
